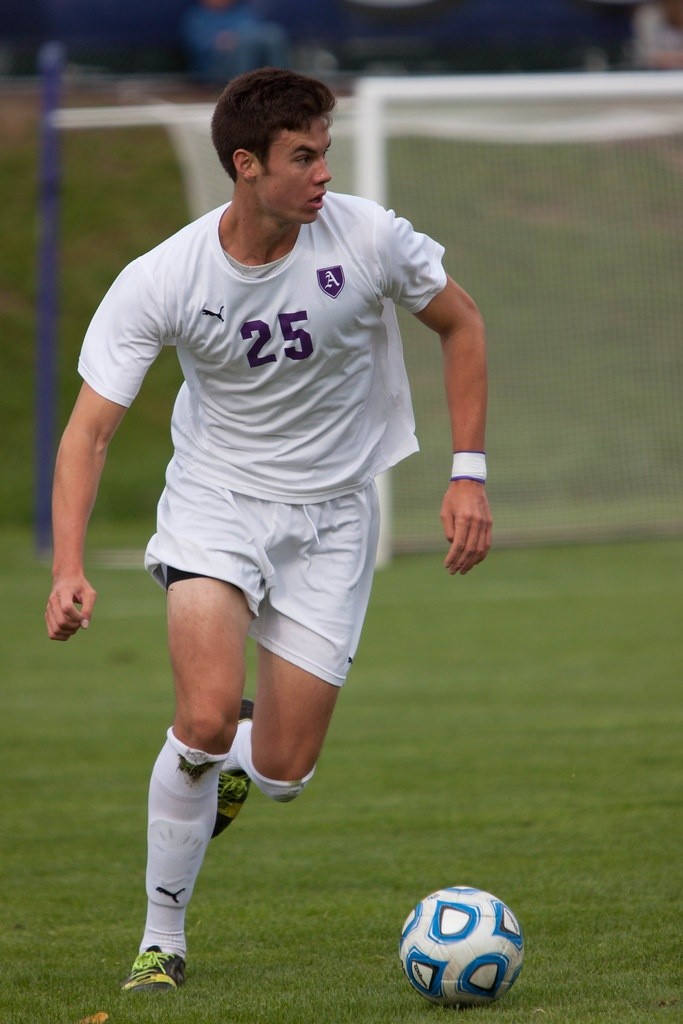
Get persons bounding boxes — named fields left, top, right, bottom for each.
left=44, top=68, right=493, bottom=997
left=627, top=0, right=680, bottom=71
left=177, top=2, right=294, bottom=95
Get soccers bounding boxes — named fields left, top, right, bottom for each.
left=397, top=885, right=525, bottom=1006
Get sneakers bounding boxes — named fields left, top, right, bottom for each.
left=210, top=699, right=255, bottom=839
left=121, top=945, right=188, bottom=995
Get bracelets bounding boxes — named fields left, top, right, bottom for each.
left=449, top=450, right=490, bottom=486
